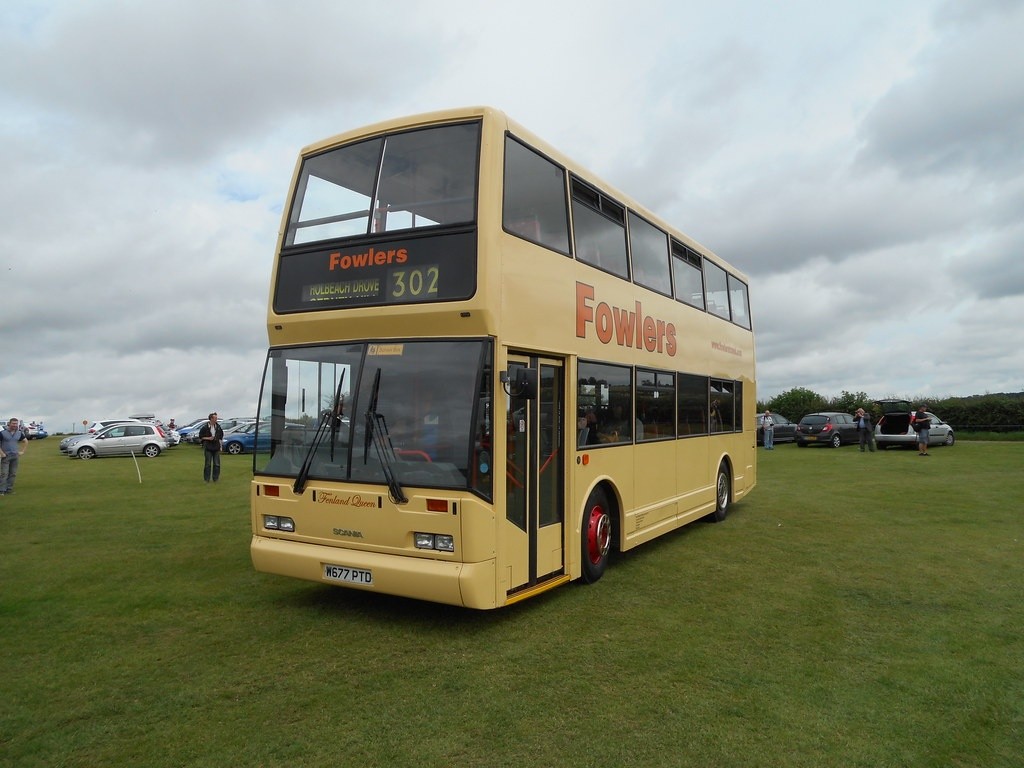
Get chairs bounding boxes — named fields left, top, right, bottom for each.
left=577, top=245, right=601, bottom=266
left=508, top=211, right=542, bottom=241
left=577, top=384, right=732, bottom=445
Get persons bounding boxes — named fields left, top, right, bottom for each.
left=0, top=418, right=29, bottom=495
left=30, top=421, right=43, bottom=430
left=914, top=404, right=932, bottom=455
left=853, top=408, right=876, bottom=452
left=338, top=369, right=408, bottom=446
left=596, top=400, right=631, bottom=443
left=199, top=412, right=223, bottom=483
left=761, top=410, right=774, bottom=450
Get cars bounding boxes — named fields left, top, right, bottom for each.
left=757, top=413, right=798, bottom=443
left=0, top=421, right=48, bottom=440
left=176, top=417, right=306, bottom=455
left=874, top=399, right=955, bottom=450
left=60, top=423, right=181, bottom=460
left=797, top=412, right=861, bottom=448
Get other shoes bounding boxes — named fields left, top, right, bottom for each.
left=765, top=447, right=774, bottom=450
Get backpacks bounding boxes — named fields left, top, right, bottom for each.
left=910, top=411, right=924, bottom=433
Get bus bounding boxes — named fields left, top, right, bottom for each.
left=250, top=105, right=757, bottom=610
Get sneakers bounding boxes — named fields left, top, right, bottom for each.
left=919, top=453, right=929, bottom=456
left=0, top=489, right=17, bottom=496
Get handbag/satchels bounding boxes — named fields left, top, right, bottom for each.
left=760, top=426, right=764, bottom=435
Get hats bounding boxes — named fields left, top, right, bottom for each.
left=919, top=404, right=928, bottom=407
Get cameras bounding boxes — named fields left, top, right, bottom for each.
left=855, top=411, right=859, bottom=416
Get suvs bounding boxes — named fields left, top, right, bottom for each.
left=88, top=419, right=141, bottom=435
